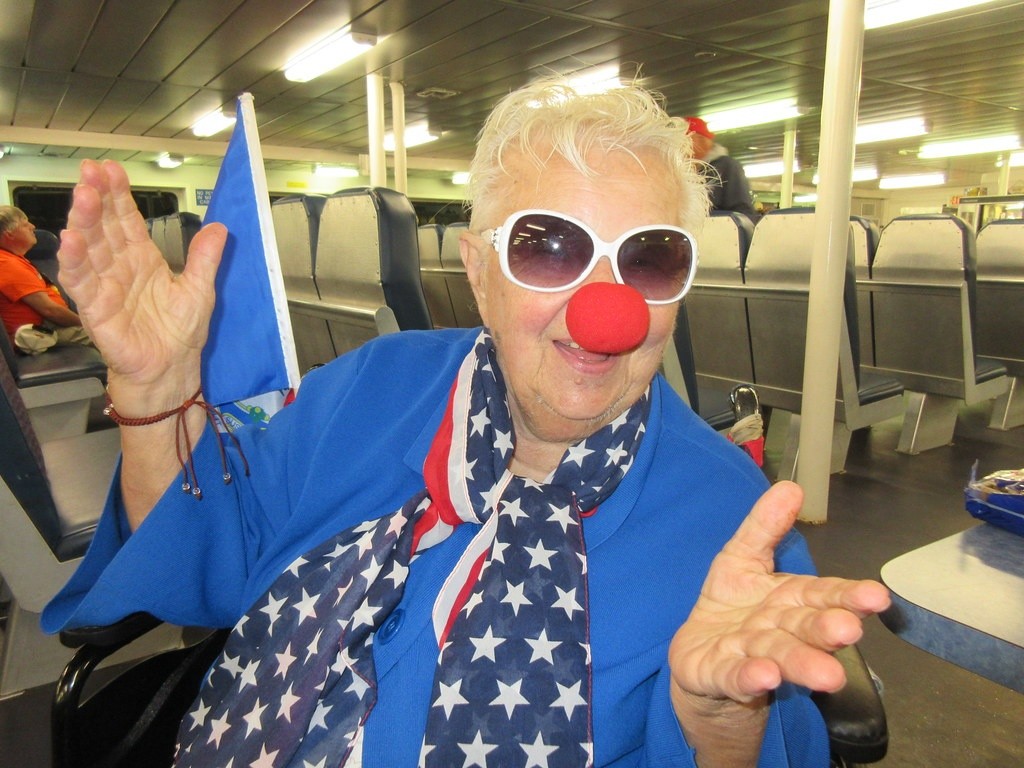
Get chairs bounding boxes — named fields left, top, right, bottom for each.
left=50, top=362, right=890, bottom=768
left=0, top=175, right=1024, bottom=613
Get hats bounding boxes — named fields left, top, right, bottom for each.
left=14, top=323, right=59, bottom=355
left=680, top=116, right=714, bottom=139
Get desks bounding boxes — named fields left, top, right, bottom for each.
left=877, top=519, right=1024, bottom=694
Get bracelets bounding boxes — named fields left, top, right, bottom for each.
left=104, top=386, right=249, bottom=497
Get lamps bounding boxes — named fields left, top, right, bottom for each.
left=812, top=169, right=881, bottom=185
left=536, top=75, right=637, bottom=108
left=383, top=121, right=442, bottom=152
left=707, top=106, right=810, bottom=134
left=285, top=32, right=377, bottom=83
left=159, top=157, right=184, bottom=169
left=855, top=125, right=933, bottom=144
left=192, top=111, right=237, bottom=137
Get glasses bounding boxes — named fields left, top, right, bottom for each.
left=478, top=209, right=699, bottom=305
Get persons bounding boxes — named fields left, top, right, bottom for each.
left=37, top=78, right=891, bottom=768
left=677, top=115, right=757, bottom=230
left=0, top=205, right=94, bottom=346
